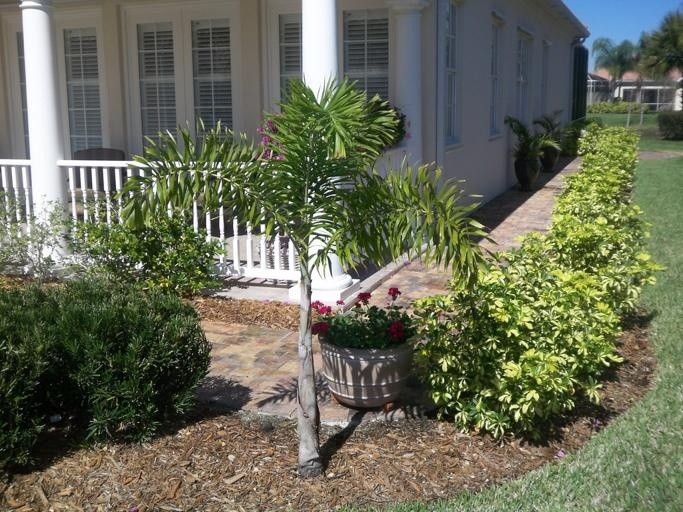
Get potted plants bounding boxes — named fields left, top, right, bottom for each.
left=503, top=111, right=573, bottom=189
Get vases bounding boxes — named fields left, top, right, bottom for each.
left=320, top=342, right=414, bottom=408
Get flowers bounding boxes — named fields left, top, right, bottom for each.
left=309, top=285, right=413, bottom=346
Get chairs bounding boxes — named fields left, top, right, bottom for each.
left=74, top=148, right=125, bottom=202
